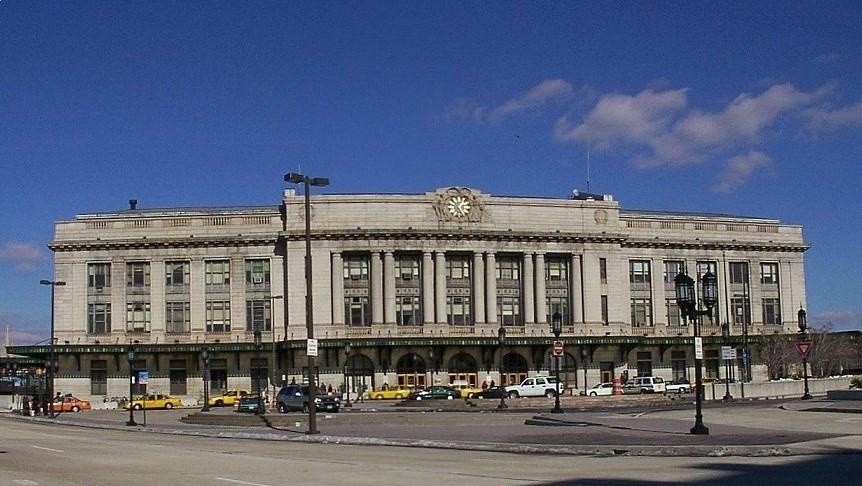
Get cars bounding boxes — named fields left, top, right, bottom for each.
left=1, top=369, right=46, bottom=388
left=122, top=393, right=183, bottom=410
left=580, top=382, right=624, bottom=396
left=691, top=378, right=720, bottom=391
left=47, top=396, right=90, bottom=413
left=362, top=383, right=509, bottom=401
left=207, top=390, right=248, bottom=405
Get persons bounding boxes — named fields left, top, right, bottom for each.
left=482, top=380, right=495, bottom=390
left=355, top=385, right=365, bottom=403
left=620, top=372, right=627, bottom=385
left=314, top=382, right=332, bottom=396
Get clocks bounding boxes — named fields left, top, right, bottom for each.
left=444, top=196, right=473, bottom=222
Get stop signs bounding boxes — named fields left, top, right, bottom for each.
left=554, top=341, right=563, bottom=356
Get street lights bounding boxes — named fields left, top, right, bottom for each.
left=127, top=344, right=139, bottom=426
left=253, top=328, right=264, bottom=416
left=428, top=346, right=436, bottom=402
left=721, top=319, right=732, bottom=400
left=795, top=303, right=812, bottom=400
left=200, top=341, right=209, bottom=413
left=344, top=341, right=353, bottom=407
left=40, top=278, right=67, bottom=417
left=284, top=170, right=330, bottom=434
left=552, top=306, right=563, bottom=413
left=582, top=347, right=588, bottom=396
left=498, top=326, right=510, bottom=409
left=675, top=264, right=715, bottom=436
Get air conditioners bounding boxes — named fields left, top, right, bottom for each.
left=254, top=276, right=262, bottom=283
left=402, top=272, right=411, bottom=279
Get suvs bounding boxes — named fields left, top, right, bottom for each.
left=666, top=380, right=690, bottom=395
left=504, top=375, right=564, bottom=399
left=276, top=385, right=340, bottom=414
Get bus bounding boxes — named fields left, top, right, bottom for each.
left=237, top=393, right=266, bottom=413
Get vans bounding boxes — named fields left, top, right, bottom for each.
left=626, top=377, right=665, bottom=393
